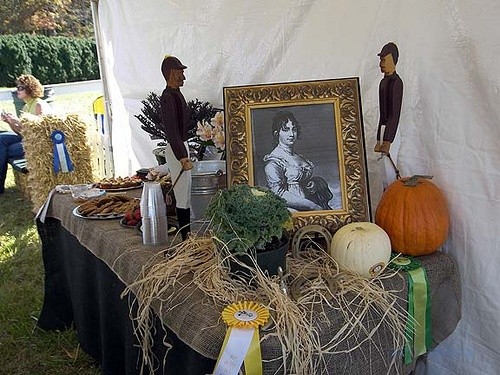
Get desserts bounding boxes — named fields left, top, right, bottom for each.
left=99, top=176, right=142, bottom=188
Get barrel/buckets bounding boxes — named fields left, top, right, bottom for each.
left=189, top=160, right=226, bottom=236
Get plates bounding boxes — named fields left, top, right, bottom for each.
left=119, top=217, right=136, bottom=228
left=88, top=183, right=144, bottom=191
left=72, top=197, right=141, bottom=219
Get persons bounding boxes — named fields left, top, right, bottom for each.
left=0, top=75, right=54, bottom=194
left=262, top=110, right=323, bottom=212
left=160, top=55, right=196, bottom=242
left=374, top=41, right=404, bottom=193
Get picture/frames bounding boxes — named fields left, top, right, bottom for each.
left=223, top=77, right=370, bottom=238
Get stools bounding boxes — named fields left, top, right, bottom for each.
left=10, top=159, right=27, bottom=192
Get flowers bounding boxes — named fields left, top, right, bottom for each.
left=195, top=111, right=225, bottom=160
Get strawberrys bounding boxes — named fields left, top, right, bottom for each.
left=123, top=207, right=141, bottom=225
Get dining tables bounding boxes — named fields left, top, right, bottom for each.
left=33, top=185, right=462, bottom=375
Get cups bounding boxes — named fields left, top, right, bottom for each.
left=139, top=182, right=169, bottom=245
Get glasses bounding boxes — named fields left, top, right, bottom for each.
left=18, top=86, right=25, bottom=91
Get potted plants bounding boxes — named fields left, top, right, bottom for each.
left=204, top=184, right=294, bottom=290
left=133, top=92, right=216, bottom=165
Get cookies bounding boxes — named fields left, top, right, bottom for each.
left=79, top=194, right=141, bottom=215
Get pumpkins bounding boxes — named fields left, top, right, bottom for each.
left=331, top=222, right=392, bottom=279
left=375, top=175, right=449, bottom=256
